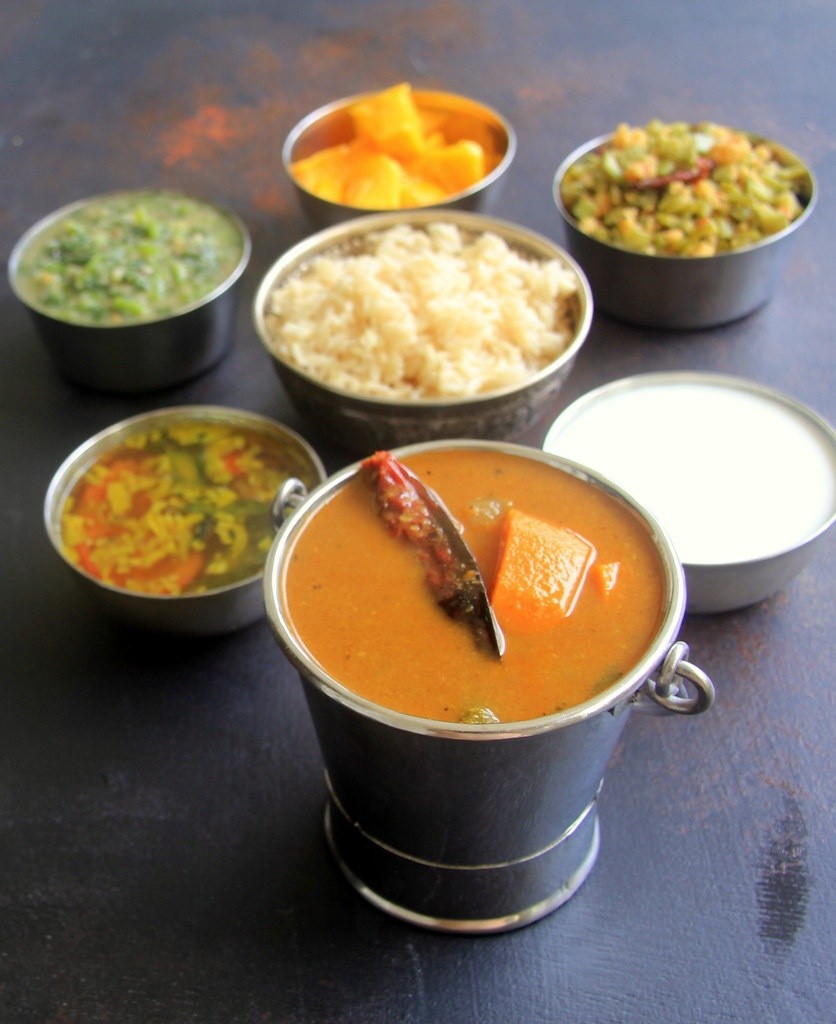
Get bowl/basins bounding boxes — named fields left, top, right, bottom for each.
left=542, top=370, right=836, bottom=615
left=280, top=92, right=517, bottom=230
left=43, top=406, right=328, bottom=633
left=6, top=188, right=253, bottom=392
left=549, top=134, right=820, bottom=330
left=254, top=209, right=593, bottom=461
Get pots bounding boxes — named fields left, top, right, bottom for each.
left=260, top=439, right=719, bottom=932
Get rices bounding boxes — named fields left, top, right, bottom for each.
left=267, top=223, right=577, bottom=400
